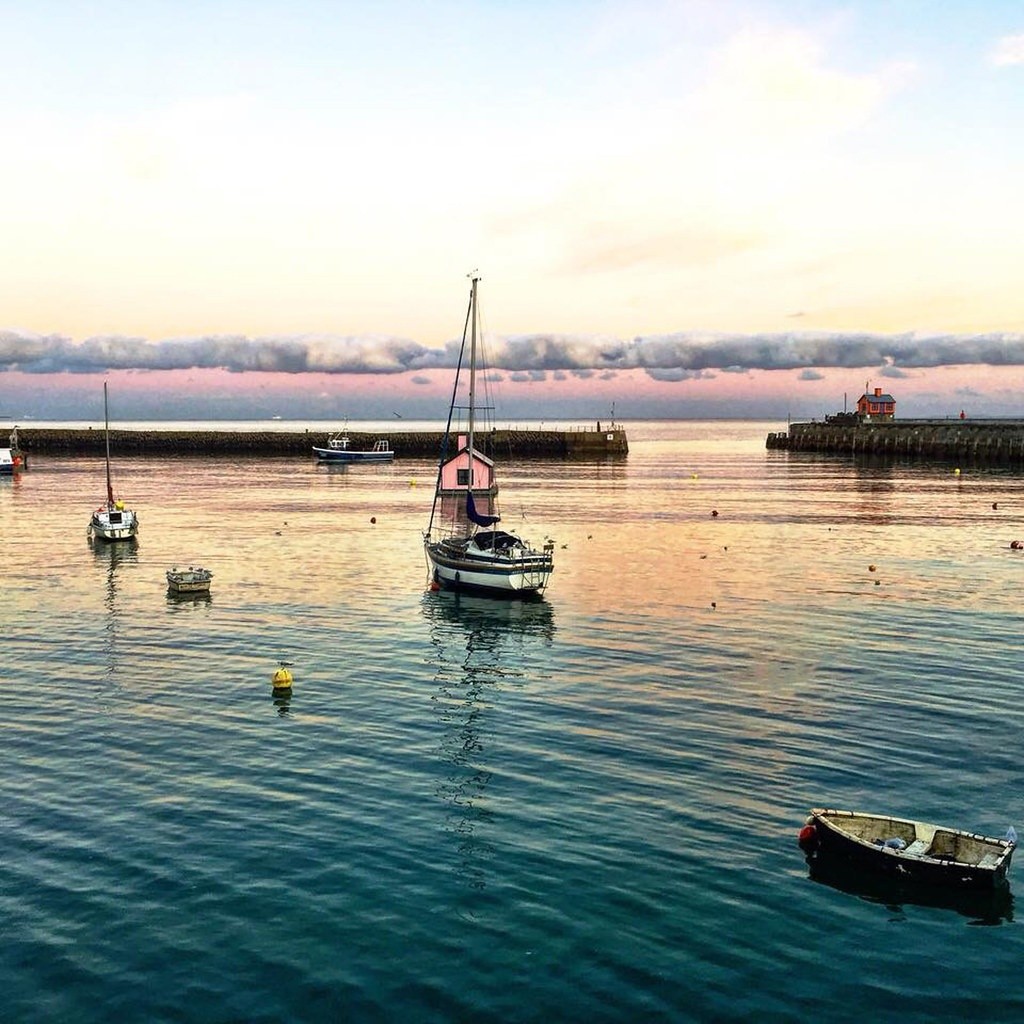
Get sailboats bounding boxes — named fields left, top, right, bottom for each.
left=90, top=379, right=139, bottom=540
left=421, top=267, right=556, bottom=593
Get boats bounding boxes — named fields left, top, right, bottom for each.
left=0, top=448, right=24, bottom=473
left=167, top=566, right=213, bottom=593
left=810, top=806, right=1017, bottom=891
left=312, top=412, right=395, bottom=461
